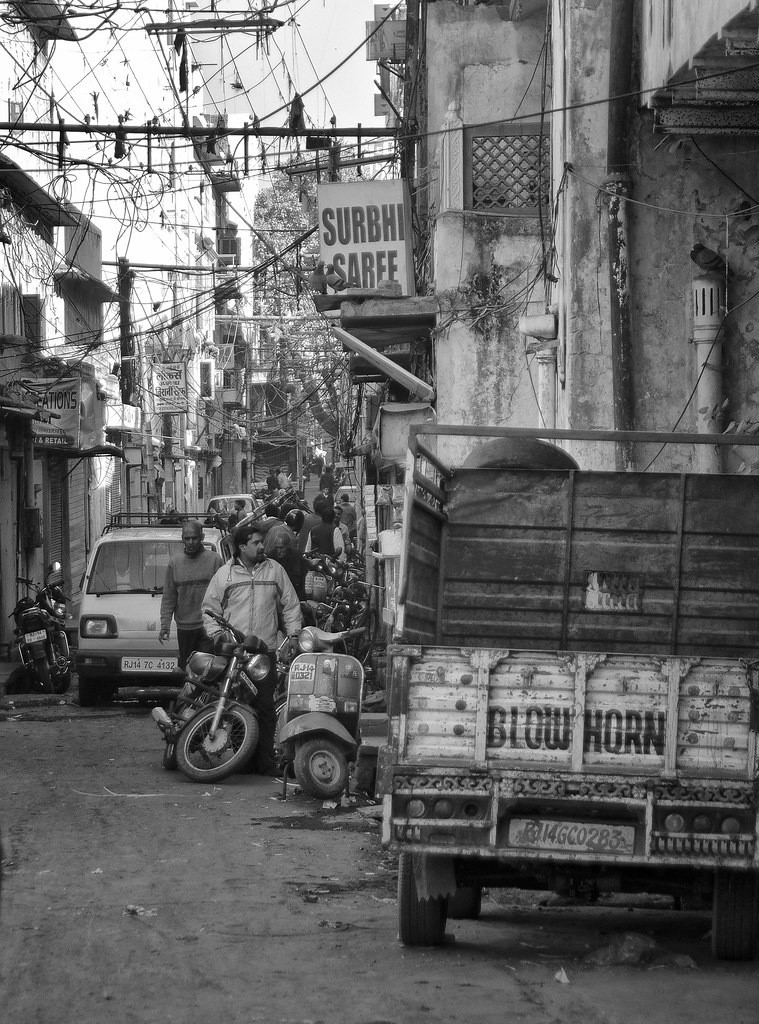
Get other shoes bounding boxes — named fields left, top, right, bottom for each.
left=243, top=750, right=284, bottom=777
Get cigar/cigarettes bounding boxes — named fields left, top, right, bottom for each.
left=263, top=553, right=267, bottom=557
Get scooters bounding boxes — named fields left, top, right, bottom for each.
left=272, top=627, right=370, bottom=799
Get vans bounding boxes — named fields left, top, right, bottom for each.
left=76, top=511, right=231, bottom=706
left=208, top=494, right=261, bottom=524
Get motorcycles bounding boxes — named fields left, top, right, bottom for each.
left=297, top=545, right=385, bottom=666
left=152, top=610, right=277, bottom=785
left=8, top=561, right=72, bottom=693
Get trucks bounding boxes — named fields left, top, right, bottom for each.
left=252, top=432, right=308, bottom=498
left=380, top=419, right=759, bottom=961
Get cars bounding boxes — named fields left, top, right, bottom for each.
left=334, top=470, right=359, bottom=506
left=256, top=499, right=266, bottom=519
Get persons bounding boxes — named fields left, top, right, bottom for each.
left=200, top=527, right=302, bottom=775
left=205, top=455, right=358, bottom=601
left=162, top=510, right=188, bottom=524
left=93, top=549, right=155, bottom=590
left=157, top=521, right=223, bottom=688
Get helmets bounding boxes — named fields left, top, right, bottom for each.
left=284, top=509, right=305, bottom=531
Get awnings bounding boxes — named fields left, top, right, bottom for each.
left=70, top=445, right=125, bottom=457
left=0, top=396, right=61, bottom=420
